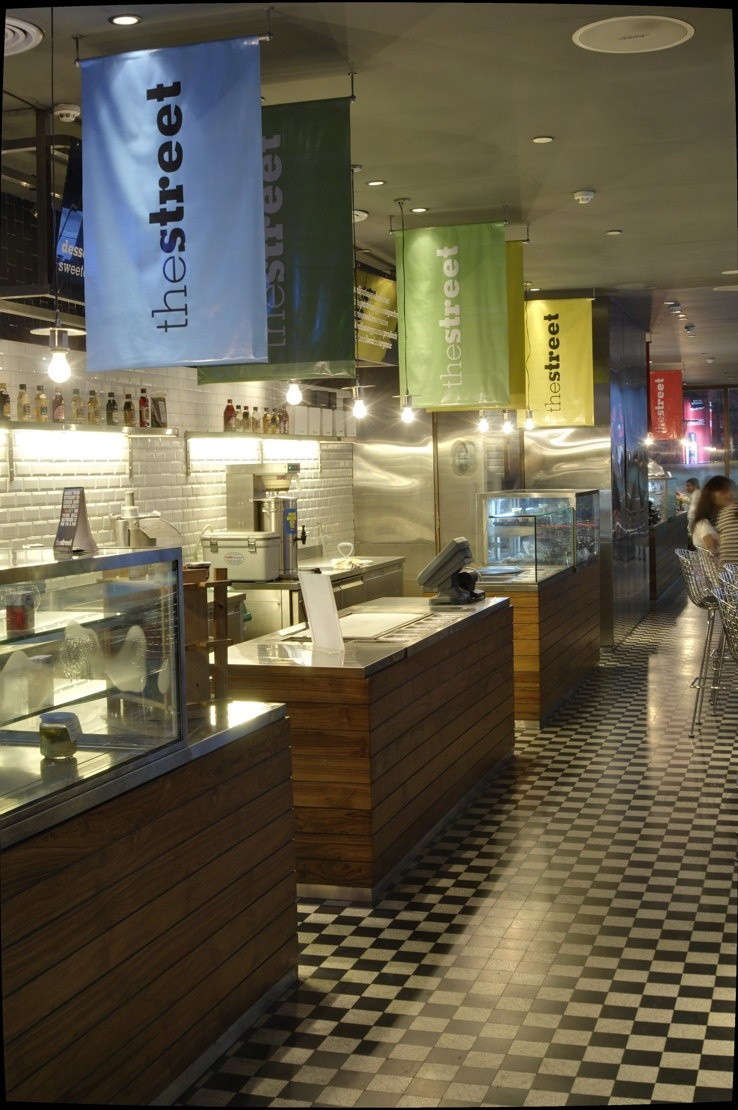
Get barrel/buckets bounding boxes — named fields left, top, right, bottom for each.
left=260, top=498, right=305, bottom=580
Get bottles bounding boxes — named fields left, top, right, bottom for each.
left=278, top=409, right=284, bottom=435
left=71, top=389, right=85, bottom=424
left=123, top=393, right=137, bottom=427
left=151, top=392, right=167, bottom=428
left=251, top=407, right=261, bottom=433
left=34, top=385, right=49, bottom=422
left=270, top=408, right=280, bottom=434
left=282, top=406, right=289, bottom=434
left=87, top=389, right=100, bottom=425
left=235, top=405, right=244, bottom=432
left=243, top=406, right=252, bottom=432
left=16, top=383, right=31, bottom=423
left=138, top=388, right=150, bottom=428
left=105, top=392, right=119, bottom=426
left=223, top=399, right=236, bottom=432
left=0, top=382, right=11, bottom=422
left=263, top=407, right=272, bottom=434
left=51, top=387, right=65, bottom=423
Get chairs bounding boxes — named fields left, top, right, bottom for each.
left=674, top=545, right=738, bottom=739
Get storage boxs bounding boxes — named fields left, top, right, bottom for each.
left=201, top=531, right=281, bottom=582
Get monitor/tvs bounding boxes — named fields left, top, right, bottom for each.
left=418, top=536, right=477, bottom=607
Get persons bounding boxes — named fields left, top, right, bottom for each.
left=674, top=478, right=701, bottom=551
left=690, top=475, right=738, bottom=564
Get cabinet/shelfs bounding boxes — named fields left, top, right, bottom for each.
left=231, top=555, right=407, bottom=643
left=422, top=553, right=601, bottom=731
left=0, top=546, right=190, bottom=824
left=649, top=511, right=688, bottom=606
left=0, top=689, right=299, bottom=1110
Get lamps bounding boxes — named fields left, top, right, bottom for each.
left=391, top=197, right=422, bottom=424
left=525, top=277, right=539, bottom=430
left=340, top=164, right=376, bottom=418
left=28, top=7, right=86, bottom=382
